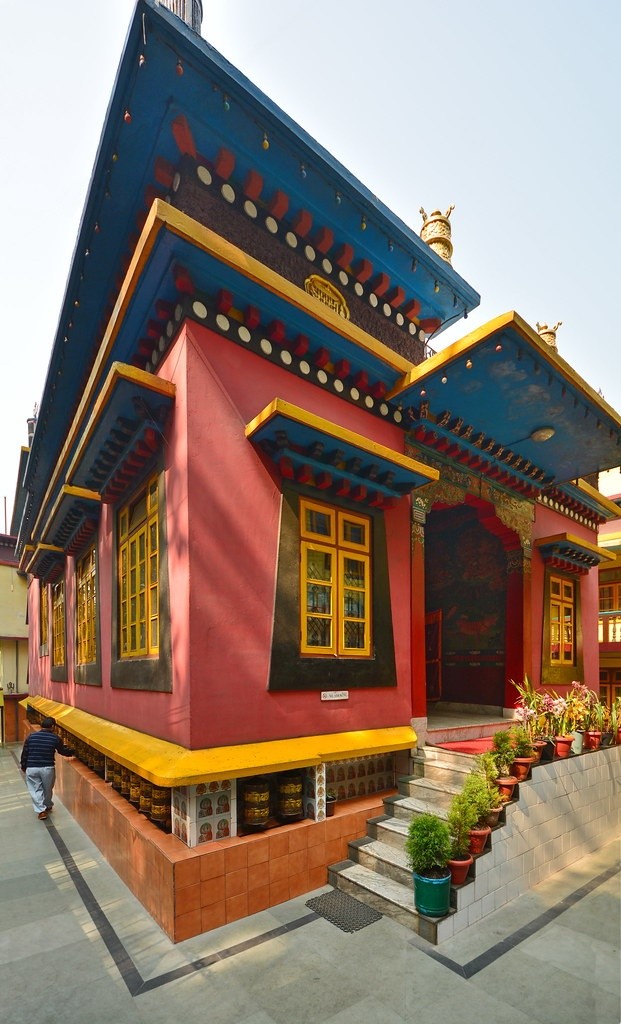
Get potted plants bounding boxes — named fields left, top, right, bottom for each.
left=404, top=726, right=537, bottom=917
left=325, top=789, right=338, bottom=816
left=584, top=691, right=621, bottom=751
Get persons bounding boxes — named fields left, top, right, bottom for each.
left=21, top=717, right=80, bottom=820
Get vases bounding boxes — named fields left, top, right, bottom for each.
left=568, top=730, right=586, bottom=754
left=538, top=738, right=555, bottom=761
left=554, top=736, right=575, bottom=758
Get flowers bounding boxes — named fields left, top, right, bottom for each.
left=509, top=673, right=597, bottom=740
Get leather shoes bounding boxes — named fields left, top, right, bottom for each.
left=46, top=806, right=53, bottom=812
left=38, top=812, right=48, bottom=819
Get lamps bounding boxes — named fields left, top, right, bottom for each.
left=532, top=428, right=554, bottom=441
